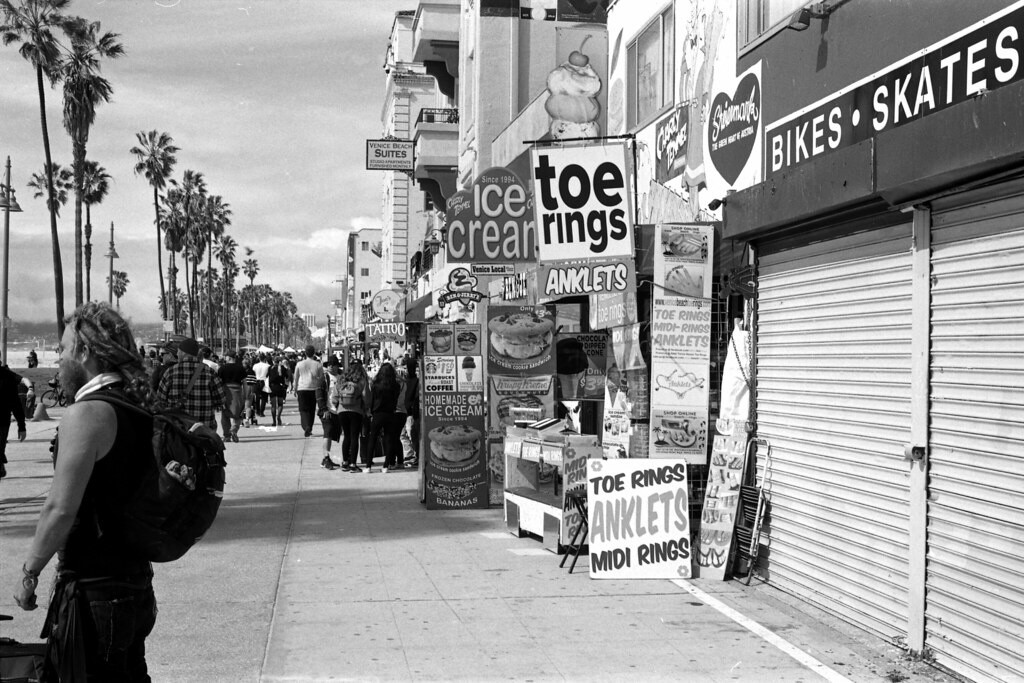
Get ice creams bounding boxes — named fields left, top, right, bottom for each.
left=462, top=357, right=475, bottom=381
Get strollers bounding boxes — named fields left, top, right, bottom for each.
left=242, top=392, right=259, bottom=425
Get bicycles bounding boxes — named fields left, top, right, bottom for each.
left=39, top=371, right=67, bottom=408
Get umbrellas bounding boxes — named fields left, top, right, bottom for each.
left=255, top=345, right=274, bottom=353
left=284, top=347, right=295, bottom=353
left=241, top=344, right=257, bottom=352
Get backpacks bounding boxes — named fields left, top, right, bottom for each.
left=338, top=379, right=361, bottom=405
left=75, top=390, right=226, bottom=562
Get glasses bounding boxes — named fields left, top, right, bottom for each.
left=161, top=352, right=169, bottom=356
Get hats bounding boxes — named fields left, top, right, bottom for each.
left=224, top=351, right=236, bottom=358
left=323, top=355, right=342, bottom=368
left=178, top=338, right=198, bottom=356
left=350, top=359, right=362, bottom=366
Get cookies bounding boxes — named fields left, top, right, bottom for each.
left=488, top=312, right=554, bottom=365
left=428, top=426, right=481, bottom=468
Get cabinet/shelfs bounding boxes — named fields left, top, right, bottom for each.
left=504, top=427, right=601, bottom=553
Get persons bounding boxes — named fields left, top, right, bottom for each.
left=12, top=302, right=157, bottom=683
left=139, top=339, right=419, bottom=473
left=0, top=349, right=38, bottom=480
left=669, top=233, right=701, bottom=255
left=671, top=267, right=703, bottom=298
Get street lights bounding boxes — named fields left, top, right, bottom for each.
left=166, top=253, right=176, bottom=345
left=102, top=221, right=119, bottom=310
left=0, top=155, right=23, bottom=368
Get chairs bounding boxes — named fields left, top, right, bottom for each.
left=730, top=437, right=770, bottom=584
left=560, top=489, right=588, bottom=573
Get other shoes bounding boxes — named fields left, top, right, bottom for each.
left=260, top=414, right=265, bottom=417
left=363, top=469, right=371, bottom=472
left=256, top=411, right=260, bottom=415
left=350, top=466, right=362, bottom=473
left=304, top=427, right=311, bottom=437
left=381, top=466, right=388, bottom=473
left=283, top=400, right=285, bottom=404
left=222, top=436, right=230, bottom=442
left=341, top=463, right=349, bottom=471
left=397, top=463, right=405, bottom=469
left=320, top=459, right=340, bottom=469
left=231, top=429, right=239, bottom=443
left=389, top=464, right=398, bottom=469
left=272, top=424, right=276, bottom=426
left=277, top=417, right=281, bottom=426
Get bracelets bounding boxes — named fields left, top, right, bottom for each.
left=22, top=563, right=40, bottom=592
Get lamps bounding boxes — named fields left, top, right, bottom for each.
left=787, top=3, right=831, bottom=31
left=708, top=197, right=727, bottom=211
left=429, top=229, right=446, bottom=254
